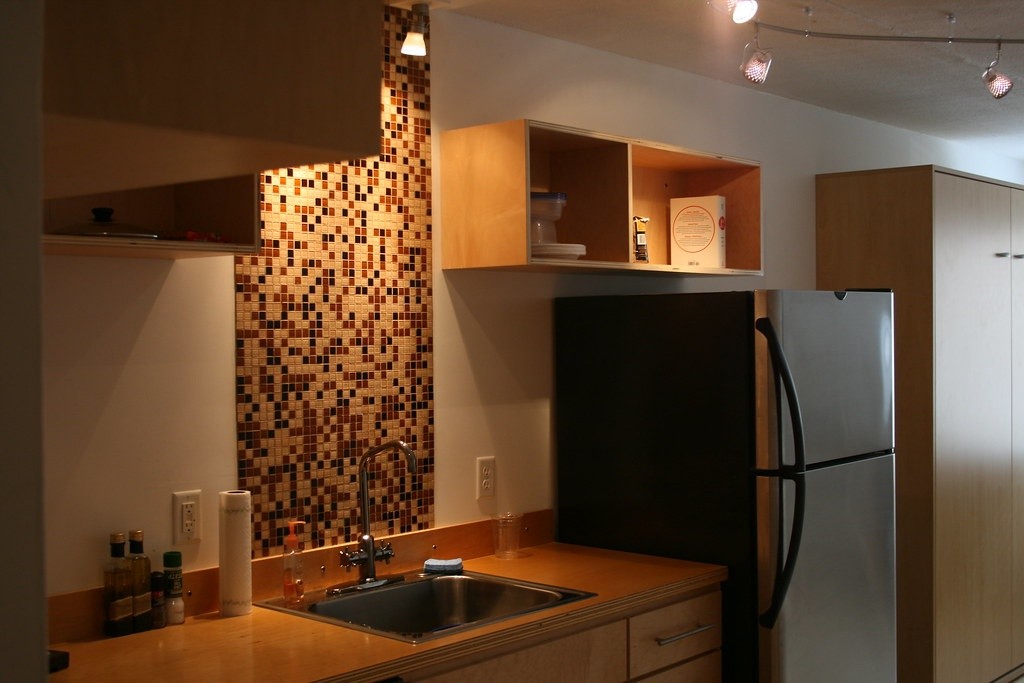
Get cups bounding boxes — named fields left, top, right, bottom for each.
left=489, top=511, right=522, bottom=559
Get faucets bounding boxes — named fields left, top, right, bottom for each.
left=358, top=440, right=417, bottom=581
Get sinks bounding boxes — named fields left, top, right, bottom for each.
left=305, top=574, right=599, bottom=644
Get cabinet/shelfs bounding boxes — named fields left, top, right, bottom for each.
left=815, top=164, right=1024, bottom=683
left=48, top=543, right=727, bottom=683
left=440, top=120, right=764, bottom=277
left=40, top=0, right=386, bottom=258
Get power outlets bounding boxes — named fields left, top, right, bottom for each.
left=476, top=457, right=496, bottom=500
left=173, top=490, right=204, bottom=547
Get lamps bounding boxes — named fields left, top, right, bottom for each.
left=401, top=5, right=429, bottom=56
left=738, top=25, right=772, bottom=85
left=721, top=0, right=758, bottom=24
left=982, top=43, right=1013, bottom=99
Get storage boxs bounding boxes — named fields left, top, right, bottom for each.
left=670, top=195, right=727, bottom=268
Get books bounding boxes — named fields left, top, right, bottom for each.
left=671, top=196, right=726, bottom=268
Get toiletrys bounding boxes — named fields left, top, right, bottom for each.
left=282, top=520, right=306, bottom=606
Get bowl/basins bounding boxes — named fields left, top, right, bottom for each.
left=530, top=191, right=567, bottom=219
left=531, top=218, right=557, bottom=244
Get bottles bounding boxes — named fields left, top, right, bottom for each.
left=103, top=529, right=185, bottom=638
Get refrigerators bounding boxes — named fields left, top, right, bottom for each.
left=550, top=287, right=898, bottom=683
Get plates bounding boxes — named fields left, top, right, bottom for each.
left=531, top=244, right=587, bottom=260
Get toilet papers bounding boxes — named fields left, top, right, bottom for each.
left=218, top=489, right=252, bottom=618
